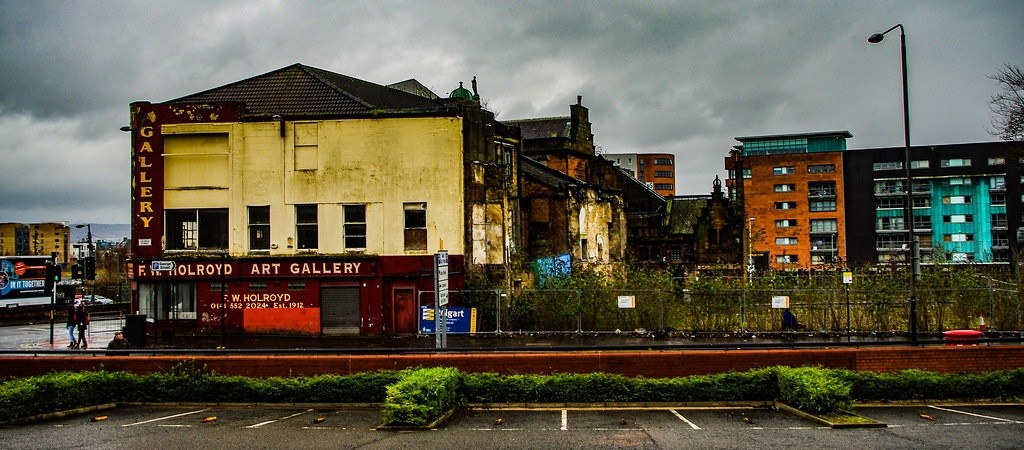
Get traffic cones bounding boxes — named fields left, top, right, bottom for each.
left=978, top=310, right=987, bottom=331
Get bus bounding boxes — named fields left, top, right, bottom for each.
left=0, top=255, right=58, bottom=297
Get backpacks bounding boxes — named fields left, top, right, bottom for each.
left=82, top=314, right=89, bottom=325
left=73, top=313, right=77, bottom=320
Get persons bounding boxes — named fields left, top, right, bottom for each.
left=105, top=331, right=132, bottom=356
left=191, top=242, right=196, bottom=250
left=66, top=301, right=90, bottom=349
left=0, top=275, right=7, bottom=287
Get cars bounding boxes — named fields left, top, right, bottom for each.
left=73, top=294, right=113, bottom=306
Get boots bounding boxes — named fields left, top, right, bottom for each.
left=73, top=340, right=76, bottom=346
left=67, top=341, right=73, bottom=347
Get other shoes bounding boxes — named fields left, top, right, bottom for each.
left=75, top=345, right=79, bottom=349
left=81, top=344, right=87, bottom=348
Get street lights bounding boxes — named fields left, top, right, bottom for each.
left=75, top=224, right=95, bottom=303
left=867, top=22, right=917, bottom=345
left=749, top=217, right=756, bottom=285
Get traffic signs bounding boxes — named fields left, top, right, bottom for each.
left=152, top=260, right=177, bottom=271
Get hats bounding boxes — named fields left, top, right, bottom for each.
left=114, top=331, right=123, bottom=336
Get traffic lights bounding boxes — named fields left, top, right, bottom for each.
left=85, top=257, right=97, bottom=280
left=53, top=266, right=61, bottom=282
